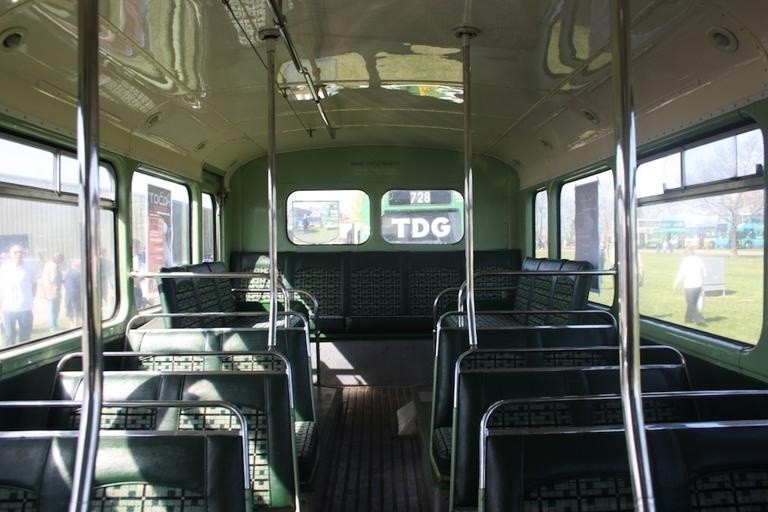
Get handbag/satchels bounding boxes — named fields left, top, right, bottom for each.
left=697, top=290, right=706, bottom=312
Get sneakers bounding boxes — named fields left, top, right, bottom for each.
left=685, top=318, right=695, bottom=323
left=697, top=320, right=709, bottom=327
left=49, top=327, right=61, bottom=333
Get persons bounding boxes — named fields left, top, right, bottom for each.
left=654, top=236, right=686, bottom=256
left=1, top=236, right=146, bottom=348
left=671, top=246, right=707, bottom=328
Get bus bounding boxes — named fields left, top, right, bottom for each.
left=599, top=221, right=765, bottom=251
left=286, top=189, right=464, bottom=245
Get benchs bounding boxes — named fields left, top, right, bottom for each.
left=159, top=261, right=320, bottom=377
left=477, top=389, right=767, bottom=510
left=1, top=400, right=251, bottom=512
left=124, top=310, right=312, bottom=459
left=51, top=350, right=301, bottom=511
left=228, top=249, right=525, bottom=335
left=448, top=345, right=691, bottom=509
left=430, top=308, right=620, bottom=480
left=431, top=258, right=594, bottom=348
